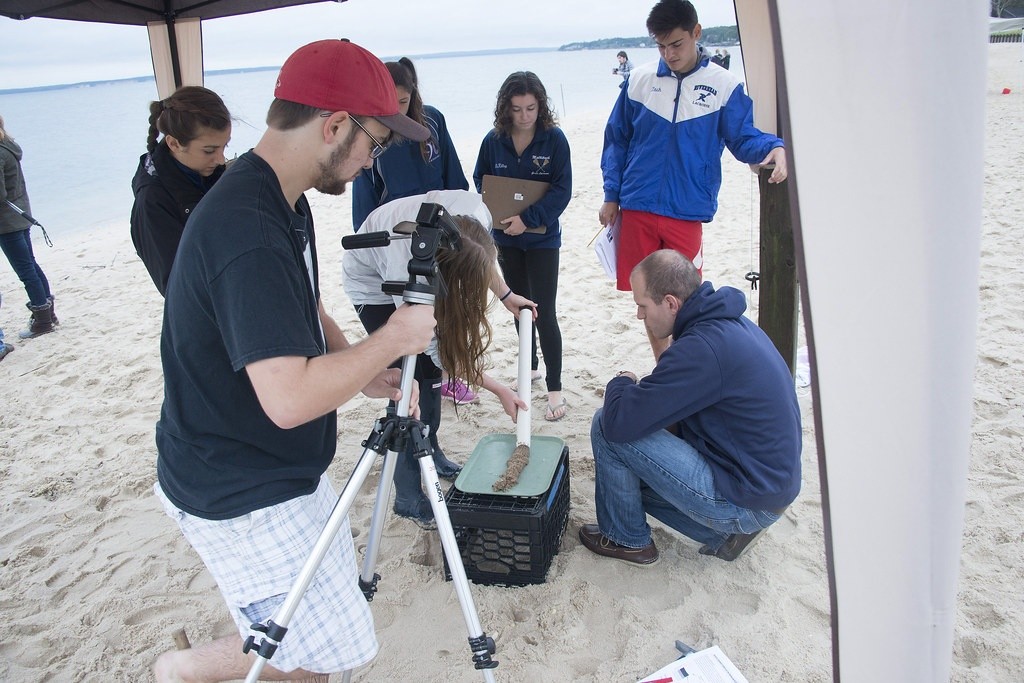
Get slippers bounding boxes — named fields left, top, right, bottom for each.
left=510, top=370, right=541, bottom=393
left=546, top=396, right=566, bottom=421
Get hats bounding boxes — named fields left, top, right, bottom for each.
left=275, top=39, right=431, bottom=143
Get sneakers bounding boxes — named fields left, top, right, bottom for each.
left=578, top=523, right=661, bottom=569
left=439, top=377, right=480, bottom=404
left=697, top=526, right=767, bottom=563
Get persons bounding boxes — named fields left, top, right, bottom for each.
left=578, top=249, right=802, bottom=569
left=710, top=49, right=722, bottom=66
left=720, top=49, right=730, bottom=69
left=0, top=115, right=60, bottom=339
left=598, top=0, right=788, bottom=365
left=129, top=87, right=231, bottom=300
left=342, top=189, right=539, bottom=532
left=613, top=50, right=634, bottom=88
left=351, top=56, right=479, bottom=404
left=154, top=37, right=437, bottom=683
left=473, top=71, right=573, bottom=421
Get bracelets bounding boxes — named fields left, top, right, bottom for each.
left=500, top=290, right=512, bottom=301
left=613, top=370, right=637, bottom=384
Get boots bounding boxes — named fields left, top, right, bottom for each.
left=47, top=295, right=59, bottom=326
left=19, top=299, right=56, bottom=339
left=419, top=375, right=463, bottom=480
left=387, top=406, right=439, bottom=530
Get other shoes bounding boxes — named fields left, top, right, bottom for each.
left=0, top=343, right=13, bottom=361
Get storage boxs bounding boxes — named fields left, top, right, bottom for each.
left=438, top=446, right=571, bottom=587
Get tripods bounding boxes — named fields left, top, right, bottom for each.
left=243, top=227, right=500, bottom=683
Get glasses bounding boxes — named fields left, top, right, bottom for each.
left=320, top=111, right=388, bottom=159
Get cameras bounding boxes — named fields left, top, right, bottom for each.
left=392, top=202, right=461, bottom=250
